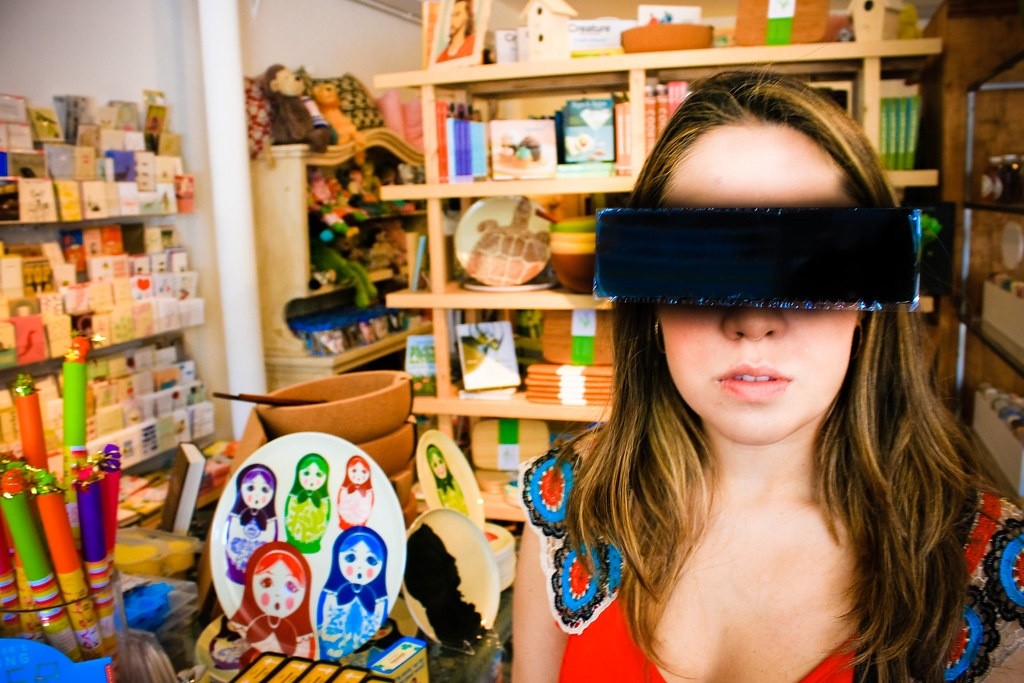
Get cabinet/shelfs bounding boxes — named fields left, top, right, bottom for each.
left=246, top=1, right=1024, bottom=523
left=0, top=213, right=214, bottom=478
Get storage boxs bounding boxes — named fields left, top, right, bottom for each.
left=972, top=391, right=1023, bottom=497
left=982, top=280, right=1024, bottom=347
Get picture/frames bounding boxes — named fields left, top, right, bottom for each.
left=431, top=1, right=490, bottom=65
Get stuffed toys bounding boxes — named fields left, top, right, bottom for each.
left=262, top=64, right=407, bottom=308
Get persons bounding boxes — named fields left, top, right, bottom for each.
left=513, top=71, right=1024, bottom=682
left=435, top=0, right=475, bottom=63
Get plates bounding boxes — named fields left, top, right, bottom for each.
left=413, top=429, right=516, bottom=593
left=404, top=509, right=501, bottom=648
left=209, top=429, right=409, bottom=663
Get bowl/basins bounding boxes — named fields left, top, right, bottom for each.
left=256, top=367, right=417, bottom=522
left=547, top=214, right=603, bottom=295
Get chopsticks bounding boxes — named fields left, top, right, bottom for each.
left=214, top=391, right=328, bottom=405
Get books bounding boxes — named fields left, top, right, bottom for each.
left=441, top=80, right=924, bottom=183
